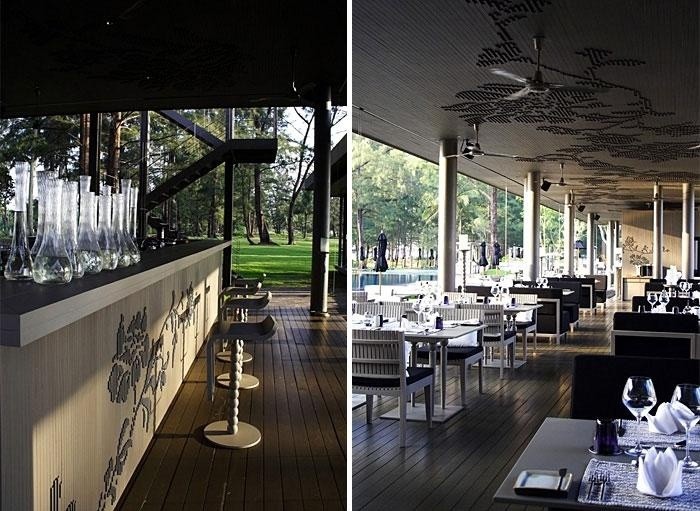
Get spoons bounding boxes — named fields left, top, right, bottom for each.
left=555, top=468, right=569, bottom=493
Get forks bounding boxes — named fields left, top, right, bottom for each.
left=586, top=470, right=608, bottom=503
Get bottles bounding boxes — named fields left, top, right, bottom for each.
left=6, top=155, right=143, bottom=286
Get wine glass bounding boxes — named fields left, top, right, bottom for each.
left=647, top=282, right=691, bottom=314
left=412, top=299, right=437, bottom=332
left=670, top=385, right=700, bottom=471
left=490, top=277, right=547, bottom=310
left=621, top=376, right=657, bottom=457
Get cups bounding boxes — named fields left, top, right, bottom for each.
left=444, top=296, right=448, bottom=305
left=365, top=311, right=373, bottom=326
left=436, top=317, right=444, bottom=329
left=594, top=416, right=619, bottom=454
left=638, top=304, right=645, bottom=313
left=484, top=297, right=489, bottom=304
left=377, top=314, right=383, bottom=327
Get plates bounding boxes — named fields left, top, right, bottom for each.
left=513, top=469, right=573, bottom=493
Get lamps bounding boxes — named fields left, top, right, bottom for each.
left=574, top=201, right=587, bottom=214
left=459, top=136, right=476, bottom=160
left=539, top=176, right=553, bottom=192
left=592, top=211, right=601, bottom=221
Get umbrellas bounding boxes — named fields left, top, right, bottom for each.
left=479, top=240, right=488, bottom=278
left=492, top=240, right=500, bottom=277
left=375, top=229, right=388, bottom=296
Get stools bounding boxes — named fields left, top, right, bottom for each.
left=194, top=263, right=280, bottom=453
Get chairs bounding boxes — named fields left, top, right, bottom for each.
left=351, top=269, right=700, bottom=510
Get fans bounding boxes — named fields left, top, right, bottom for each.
left=488, top=33, right=602, bottom=107
left=538, top=161, right=593, bottom=189
left=441, top=120, right=528, bottom=166
